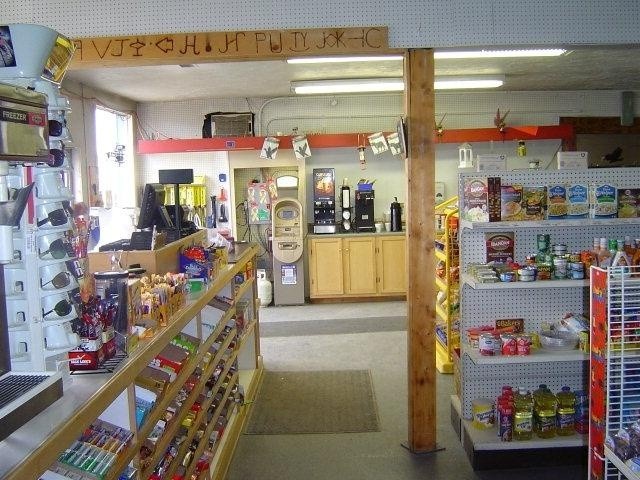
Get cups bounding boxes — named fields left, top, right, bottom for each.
left=384, top=222, right=392, bottom=232
left=375, top=223, right=382, bottom=233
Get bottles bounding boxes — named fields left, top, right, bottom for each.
left=496, top=383, right=576, bottom=443
left=593, top=235, right=640, bottom=280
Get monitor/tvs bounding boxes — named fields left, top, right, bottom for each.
left=136, top=182, right=175, bottom=230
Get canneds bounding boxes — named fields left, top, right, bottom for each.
left=517, top=234, right=585, bottom=282
left=478, top=333, right=532, bottom=357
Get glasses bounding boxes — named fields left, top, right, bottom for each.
left=41, top=239, right=66, bottom=261
left=43, top=297, right=72, bottom=318
left=49, top=120, right=63, bottom=137
left=42, top=271, right=71, bottom=290
left=37, top=209, right=68, bottom=228
left=49, top=148, right=66, bottom=167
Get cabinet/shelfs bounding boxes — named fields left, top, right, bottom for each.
left=309, top=235, right=409, bottom=302
left=0, top=241, right=267, bottom=480
left=436, top=168, right=640, bottom=479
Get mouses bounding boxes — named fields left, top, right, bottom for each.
left=120, top=242, right=134, bottom=250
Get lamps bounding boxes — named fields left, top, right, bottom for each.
left=288, top=74, right=506, bottom=97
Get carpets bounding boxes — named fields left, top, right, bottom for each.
left=244, top=368, right=385, bottom=437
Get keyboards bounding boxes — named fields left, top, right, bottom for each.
left=99, top=238, right=131, bottom=252
